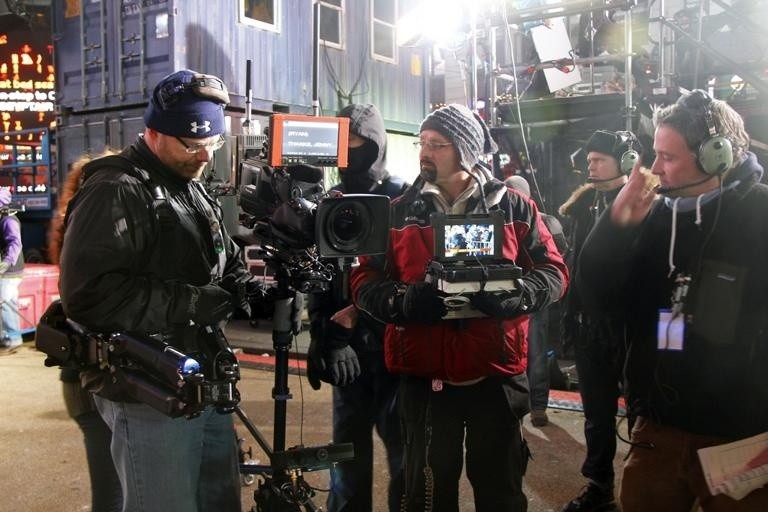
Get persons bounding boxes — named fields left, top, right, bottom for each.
left=309, top=102, right=414, bottom=511
left=1, top=185, right=24, bottom=356
left=502, top=174, right=569, bottom=427
left=574, top=88, right=768, bottom=512
left=57, top=68, right=249, bottom=512
left=552, top=127, right=666, bottom=512
left=351, top=102, right=569, bottom=512
left=48, top=143, right=124, bottom=512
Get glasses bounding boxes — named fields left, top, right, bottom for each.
left=173, top=136, right=225, bottom=155
left=412, top=140, right=454, bottom=152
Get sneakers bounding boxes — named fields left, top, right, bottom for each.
left=0, top=337, right=24, bottom=355
left=562, top=484, right=614, bottom=511
left=531, top=409, right=547, bottom=426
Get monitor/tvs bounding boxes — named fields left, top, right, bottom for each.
left=268, top=114, right=350, bottom=168
left=430, top=209, right=505, bottom=263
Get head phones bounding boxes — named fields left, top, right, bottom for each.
left=687, top=90, right=733, bottom=176
left=156, top=73, right=230, bottom=111
left=620, top=131, right=640, bottom=175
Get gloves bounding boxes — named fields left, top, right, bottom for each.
left=196, top=268, right=254, bottom=322
left=405, top=282, right=448, bottom=324
left=327, top=344, right=362, bottom=388
left=475, top=284, right=526, bottom=319
left=0, top=261, right=10, bottom=274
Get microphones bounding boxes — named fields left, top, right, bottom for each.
left=587, top=169, right=632, bottom=183
left=655, top=163, right=727, bottom=194
left=457, top=161, right=489, bottom=214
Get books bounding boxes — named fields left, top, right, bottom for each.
left=697, top=429, right=767, bottom=501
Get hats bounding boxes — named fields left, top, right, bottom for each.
left=0, top=188, right=13, bottom=206
left=586, top=129, right=641, bottom=161
left=421, top=103, right=498, bottom=172
left=144, top=68, right=226, bottom=138
left=503, top=175, right=531, bottom=200
